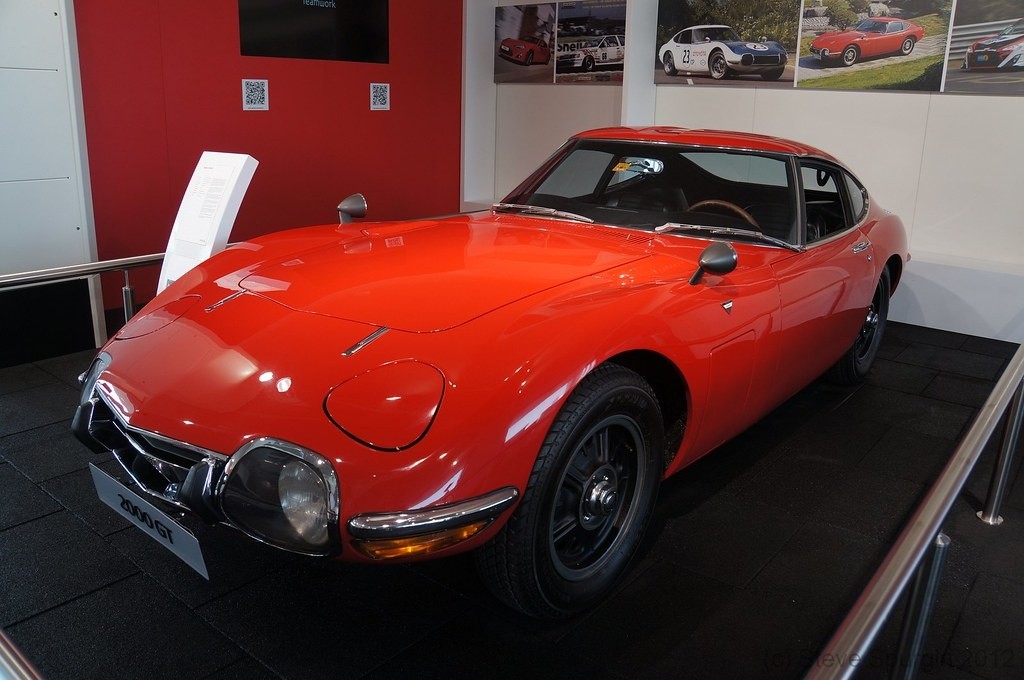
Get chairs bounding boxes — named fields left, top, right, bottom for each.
left=752, top=202, right=827, bottom=245
left=617, top=176, right=689, bottom=213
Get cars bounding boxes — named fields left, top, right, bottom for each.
left=71, top=125, right=913, bottom=622
left=568, top=34, right=624, bottom=71
left=658, top=25, right=788, bottom=81
left=962, top=19, right=1024, bottom=70
left=809, top=16, right=926, bottom=67
left=498, top=34, right=551, bottom=66
